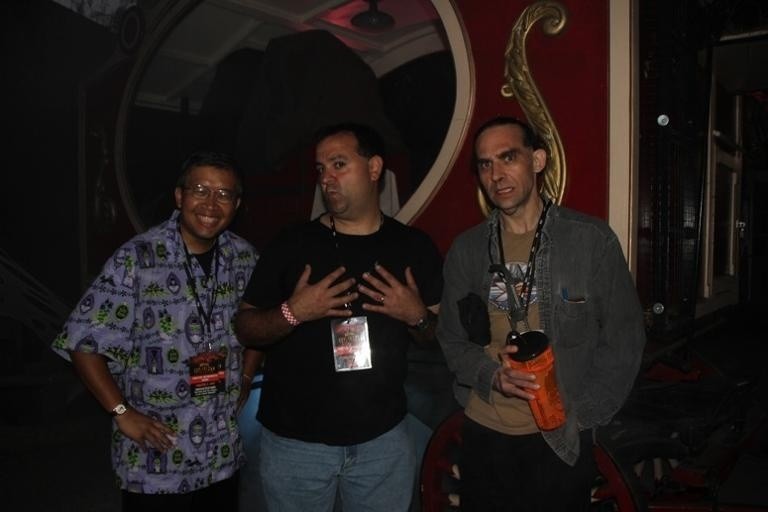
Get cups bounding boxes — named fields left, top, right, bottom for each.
left=506, top=331, right=567, bottom=431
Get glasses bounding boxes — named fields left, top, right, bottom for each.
left=183, top=184, right=237, bottom=205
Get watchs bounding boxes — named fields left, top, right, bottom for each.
left=109, top=399, right=128, bottom=417
left=404, top=309, right=433, bottom=330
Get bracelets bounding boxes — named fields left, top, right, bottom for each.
left=243, top=373, right=253, bottom=381
left=280, top=300, right=300, bottom=327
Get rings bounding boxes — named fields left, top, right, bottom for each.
left=380, top=295, right=385, bottom=303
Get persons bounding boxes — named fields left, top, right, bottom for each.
left=433, top=116, right=648, bottom=512
left=234, top=122, right=443, bottom=512
left=49, top=149, right=261, bottom=512
